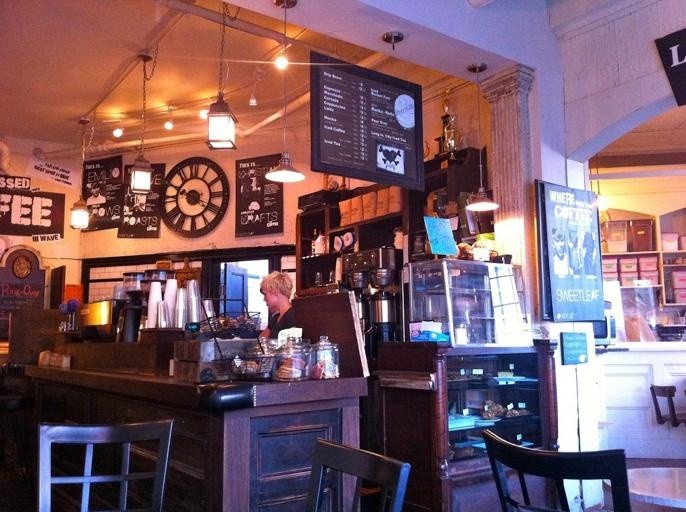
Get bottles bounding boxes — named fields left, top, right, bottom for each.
left=413, top=235, right=424, bottom=253
left=311, top=228, right=318, bottom=254
left=247, top=336, right=340, bottom=382
left=315, top=229, right=325, bottom=254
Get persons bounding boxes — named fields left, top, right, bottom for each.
left=255, top=270, right=296, bottom=338
left=85, top=181, right=107, bottom=205
left=550, top=206, right=597, bottom=281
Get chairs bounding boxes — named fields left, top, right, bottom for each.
left=36, top=418, right=175, bottom=512
left=482, top=429, right=631, bottom=512
left=306, top=437, right=411, bottom=511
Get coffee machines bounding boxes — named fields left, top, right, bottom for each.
left=110, top=269, right=177, bottom=342
left=342, top=248, right=403, bottom=358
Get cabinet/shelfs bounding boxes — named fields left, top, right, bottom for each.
left=597, top=206, right=664, bottom=308
left=657, top=206, right=686, bottom=308
left=297, top=147, right=488, bottom=343
left=373, top=343, right=557, bottom=512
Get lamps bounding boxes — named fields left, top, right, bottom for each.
left=594, top=153, right=608, bottom=211
left=208, top=1, right=238, bottom=151
left=68, top=117, right=89, bottom=230
left=264, top=0, right=305, bottom=187
left=128, top=54, right=154, bottom=194
left=464, top=62, right=499, bottom=213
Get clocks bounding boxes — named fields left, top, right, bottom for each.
left=160, top=157, right=230, bottom=237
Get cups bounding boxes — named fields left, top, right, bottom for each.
left=147, top=279, right=218, bottom=330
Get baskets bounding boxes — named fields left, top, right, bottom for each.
left=211, top=356, right=279, bottom=382
left=199, top=312, right=260, bottom=339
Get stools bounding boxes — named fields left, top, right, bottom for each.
left=1, top=397, right=34, bottom=490
